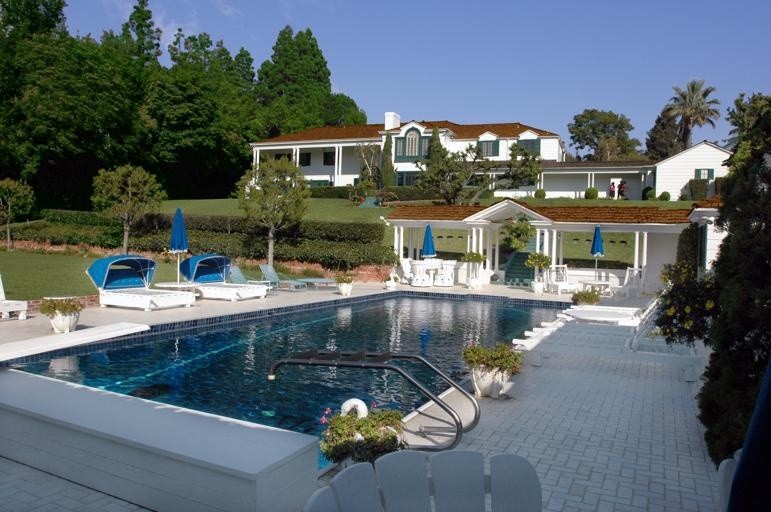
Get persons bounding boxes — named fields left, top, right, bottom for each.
left=618, top=181, right=623, bottom=199
left=622, top=181, right=629, bottom=200
left=609, top=182, right=615, bottom=199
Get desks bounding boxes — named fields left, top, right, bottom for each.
left=578, top=280, right=609, bottom=297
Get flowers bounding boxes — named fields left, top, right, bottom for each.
left=316, top=402, right=404, bottom=469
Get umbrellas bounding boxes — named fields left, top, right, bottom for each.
left=590, top=225, right=605, bottom=282
left=167, top=208, right=189, bottom=285
left=168, top=336, right=184, bottom=401
left=419, top=327, right=431, bottom=354
left=420, top=224, right=437, bottom=258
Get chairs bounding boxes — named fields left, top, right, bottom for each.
left=85, top=254, right=308, bottom=312
left=609, top=265, right=646, bottom=300
left=547, top=264, right=575, bottom=294
left=400, top=255, right=456, bottom=287
left=0, top=273, right=29, bottom=319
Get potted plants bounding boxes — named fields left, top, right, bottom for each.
left=336, top=272, right=353, bottom=296
left=463, top=335, right=521, bottom=399
left=462, top=251, right=487, bottom=290
left=39, top=298, right=84, bottom=333
left=524, top=251, right=551, bottom=292
left=573, top=285, right=602, bottom=305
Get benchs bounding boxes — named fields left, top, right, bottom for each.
left=297, top=278, right=338, bottom=289
